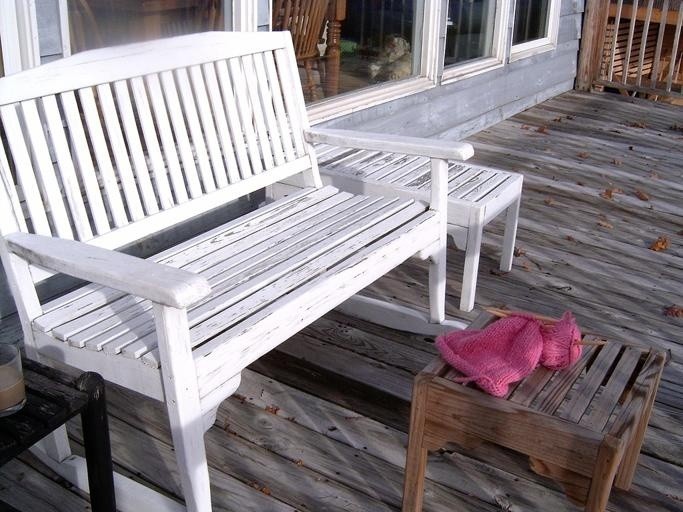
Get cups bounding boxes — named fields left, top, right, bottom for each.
left=0, top=342, right=26, bottom=417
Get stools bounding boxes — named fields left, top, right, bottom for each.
left=402, top=305, right=668, bottom=512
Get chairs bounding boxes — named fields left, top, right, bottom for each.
left=272, top=1, right=335, bottom=103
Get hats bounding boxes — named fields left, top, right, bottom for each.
left=435, top=317, right=543, bottom=397
